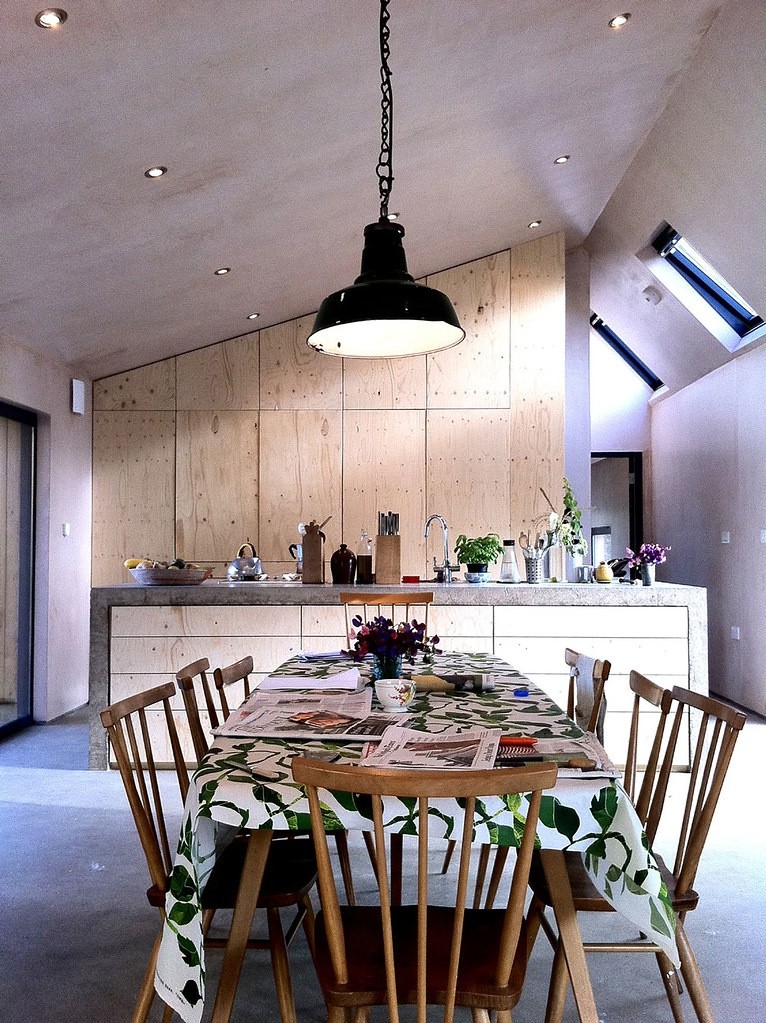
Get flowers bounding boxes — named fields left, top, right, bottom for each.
left=339, top=615, right=442, bottom=678
left=626, top=543, right=671, bottom=568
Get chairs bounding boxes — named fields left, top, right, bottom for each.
left=99, top=592, right=746, bottom=1023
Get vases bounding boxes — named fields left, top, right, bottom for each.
left=641, top=564, right=655, bottom=585
left=545, top=532, right=565, bottom=582
left=373, top=654, right=402, bottom=686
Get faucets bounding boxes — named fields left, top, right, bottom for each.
left=423, top=513, right=461, bottom=584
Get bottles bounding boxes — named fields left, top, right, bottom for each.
left=330, top=543, right=357, bottom=585
left=356, top=532, right=374, bottom=584
left=499, top=540, right=522, bottom=584
left=595, top=561, right=614, bottom=584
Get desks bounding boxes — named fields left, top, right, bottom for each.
left=154, top=651, right=683, bottom=1023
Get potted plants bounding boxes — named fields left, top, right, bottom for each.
left=454, top=532, right=505, bottom=572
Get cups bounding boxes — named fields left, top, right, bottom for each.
left=288, top=543, right=304, bottom=574
left=575, top=565, right=595, bottom=584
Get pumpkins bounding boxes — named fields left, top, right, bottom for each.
left=596, top=562, right=614, bottom=581
left=124, top=558, right=188, bottom=569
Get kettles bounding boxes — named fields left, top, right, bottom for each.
left=224, top=542, right=265, bottom=581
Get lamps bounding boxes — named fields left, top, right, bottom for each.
left=305, top=0, right=467, bottom=361
left=72, top=379, right=85, bottom=415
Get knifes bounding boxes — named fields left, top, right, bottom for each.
left=376, top=510, right=400, bottom=535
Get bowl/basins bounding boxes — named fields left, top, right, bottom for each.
left=464, top=573, right=492, bottom=583
left=374, top=678, right=417, bottom=713
left=129, top=568, right=207, bottom=585
